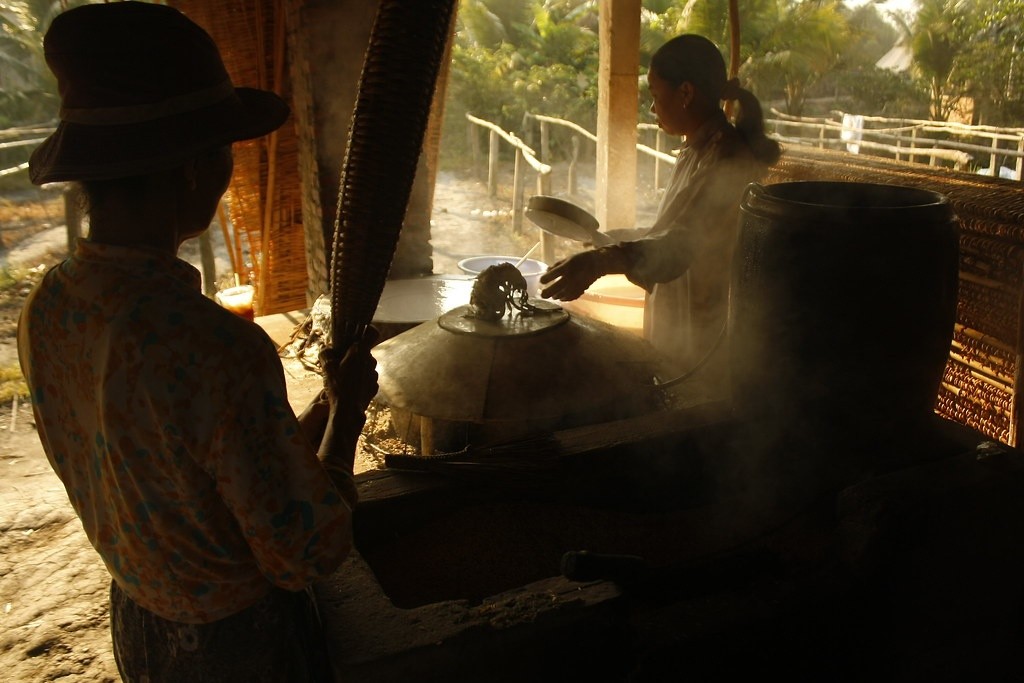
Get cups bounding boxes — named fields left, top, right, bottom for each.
left=216, top=285, right=256, bottom=322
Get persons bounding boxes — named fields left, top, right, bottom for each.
left=14, top=0, right=379, bottom=683
left=538, top=35, right=780, bottom=414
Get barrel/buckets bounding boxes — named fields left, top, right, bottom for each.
left=724, top=180, right=962, bottom=436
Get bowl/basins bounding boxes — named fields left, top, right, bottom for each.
left=457, top=255, right=548, bottom=296
left=554, top=267, right=647, bottom=329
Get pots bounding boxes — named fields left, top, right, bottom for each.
left=368, top=295, right=660, bottom=451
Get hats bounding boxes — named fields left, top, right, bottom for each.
left=29, top=0, right=291, bottom=185
